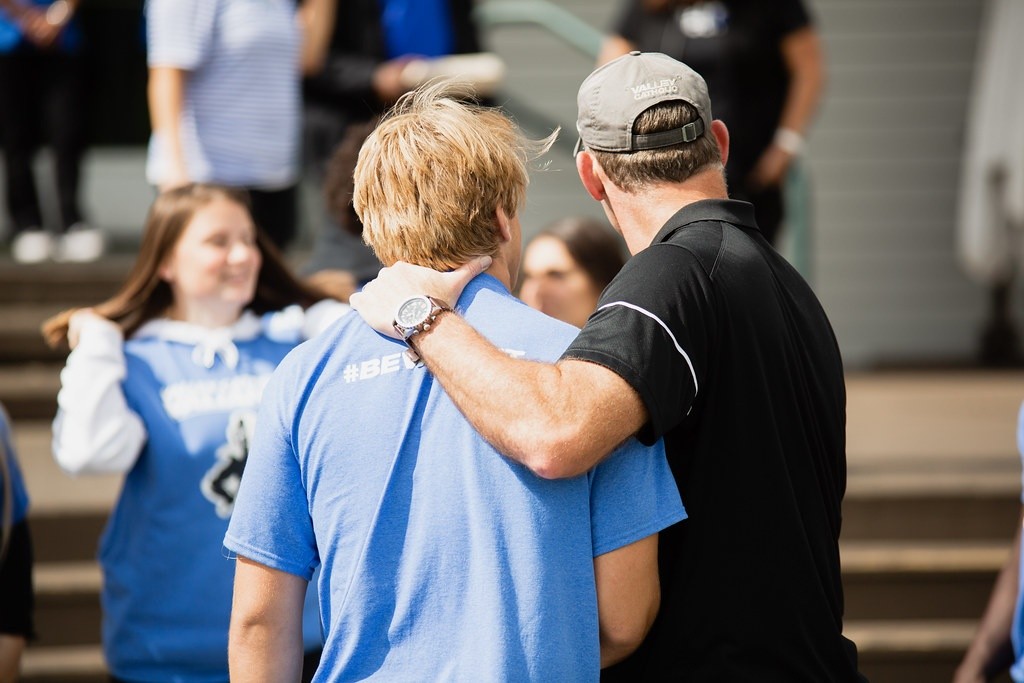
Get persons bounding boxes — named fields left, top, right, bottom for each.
left=40, top=181, right=295, bottom=682
left=223, top=77, right=692, bottom=683
left=596, top=0, right=822, bottom=252
left=304, top=0, right=497, bottom=291
left=0, top=0, right=105, bottom=262
left=954, top=0, right=1024, bottom=370
left=0, top=405, right=33, bottom=683
left=954, top=406, right=1024, bottom=683
left=349, top=53, right=860, bottom=682
left=517, top=219, right=626, bottom=328
left=145, top=0, right=335, bottom=314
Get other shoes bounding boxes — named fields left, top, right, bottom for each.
left=63, top=226, right=104, bottom=262
left=13, top=230, right=46, bottom=265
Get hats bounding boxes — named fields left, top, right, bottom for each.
left=573, top=50, right=712, bottom=157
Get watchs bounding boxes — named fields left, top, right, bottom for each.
left=393, top=296, right=457, bottom=362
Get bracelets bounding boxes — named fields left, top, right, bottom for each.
left=773, top=127, right=804, bottom=154
